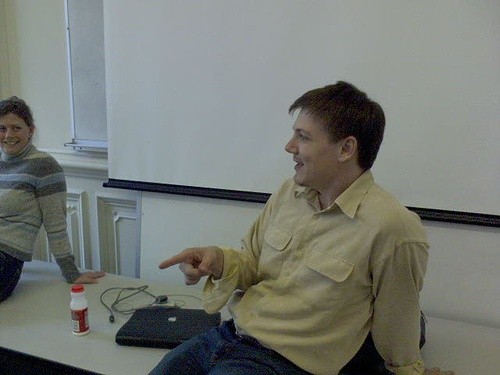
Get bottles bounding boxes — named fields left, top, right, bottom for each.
left=70, top=284, right=91, bottom=336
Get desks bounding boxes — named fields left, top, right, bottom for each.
left=0, top=259, right=500, bottom=375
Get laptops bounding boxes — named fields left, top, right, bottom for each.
left=116, top=307, right=221, bottom=349
